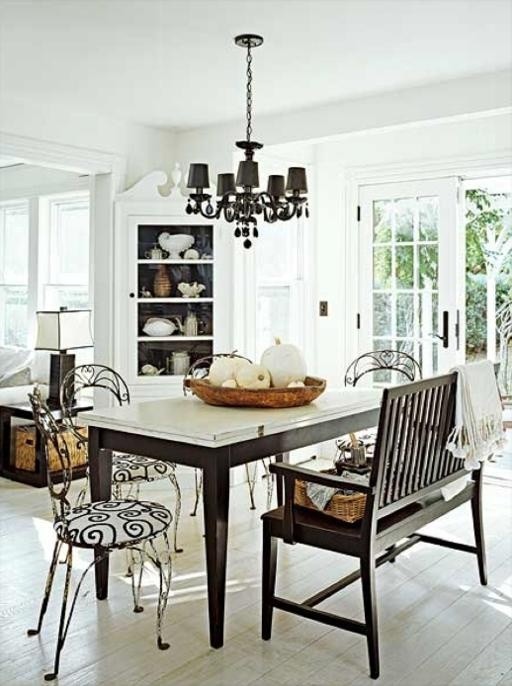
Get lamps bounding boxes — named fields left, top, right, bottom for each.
left=34, top=307, right=96, bottom=405
left=185, top=34, right=309, bottom=249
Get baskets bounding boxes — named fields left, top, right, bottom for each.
left=294, top=479, right=367, bottom=523
left=190, top=376, right=325, bottom=408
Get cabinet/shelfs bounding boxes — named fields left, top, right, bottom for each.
left=115, top=161, right=225, bottom=384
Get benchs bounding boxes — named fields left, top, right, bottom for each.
left=261, top=361, right=501, bottom=680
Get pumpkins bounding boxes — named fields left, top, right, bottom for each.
left=208, top=339, right=306, bottom=388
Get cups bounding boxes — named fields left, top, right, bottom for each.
left=143, top=243, right=168, bottom=259
left=351, top=445, right=368, bottom=468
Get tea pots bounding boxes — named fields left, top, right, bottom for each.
left=174, top=311, right=197, bottom=336
left=167, top=348, right=191, bottom=375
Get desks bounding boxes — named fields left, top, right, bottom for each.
left=1, top=400, right=93, bottom=488
